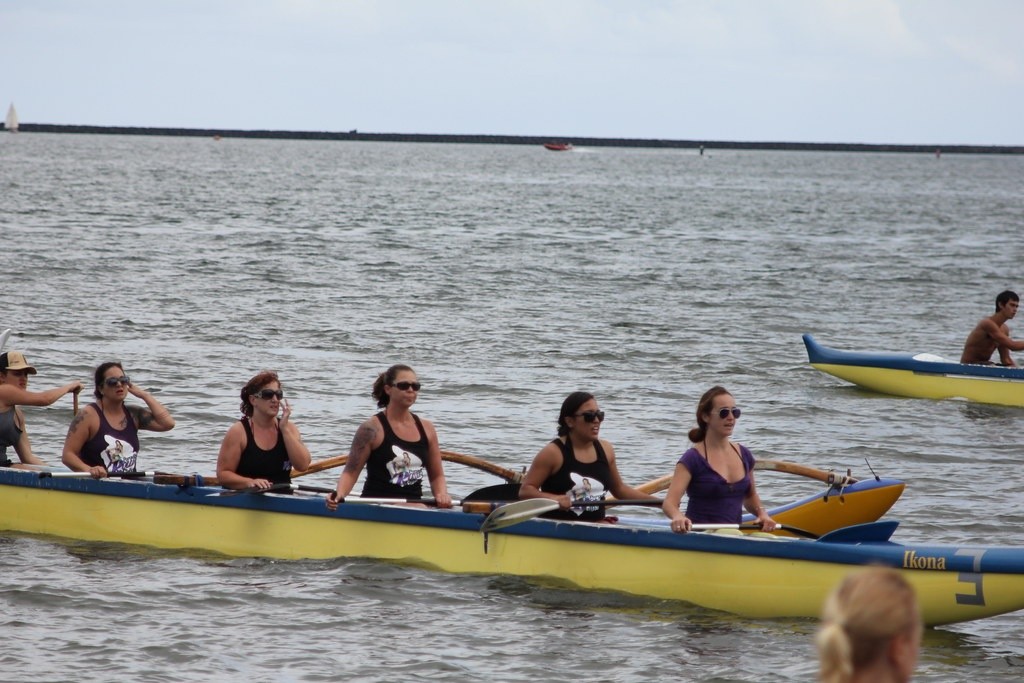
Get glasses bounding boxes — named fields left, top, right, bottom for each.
left=254, top=389, right=283, bottom=400
left=575, top=411, right=604, bottom=423
left=392, top=381, right=421, bottom=390
left=106, top=377, right=131, bottom=387
left=719, top=408, right=741, bottom=419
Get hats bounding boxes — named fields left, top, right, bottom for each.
left=0, top=351, right=37, bottom=375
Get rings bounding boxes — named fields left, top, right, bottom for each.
left=676, top=526, right=680, bottom=528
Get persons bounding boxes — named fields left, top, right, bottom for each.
left=662, top=386, right=776, bottom=534
left=63, top=362, right=175, bottom=479
left=814, top=565, right=923, bottom=683
left=215, top=370, right=311, bottom=496
left=0, top=351, right=84, bottom=470
left=962, top=291, right=1024, bottom=366
left=519, top=392, right=680, bottom=524
left=326, top=365, right=452, bottom=510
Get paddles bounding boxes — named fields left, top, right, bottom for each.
left=70, top=388, right=82, bottom=416
left=204, top=483, right=334, bottom=499
left=477, top=494, right=665, bottom=535
left=37, top=470, right=174, bottom=480
left=688, top=515, right=818, bottom=537
left=328, top=492, right=466, bottom=506
